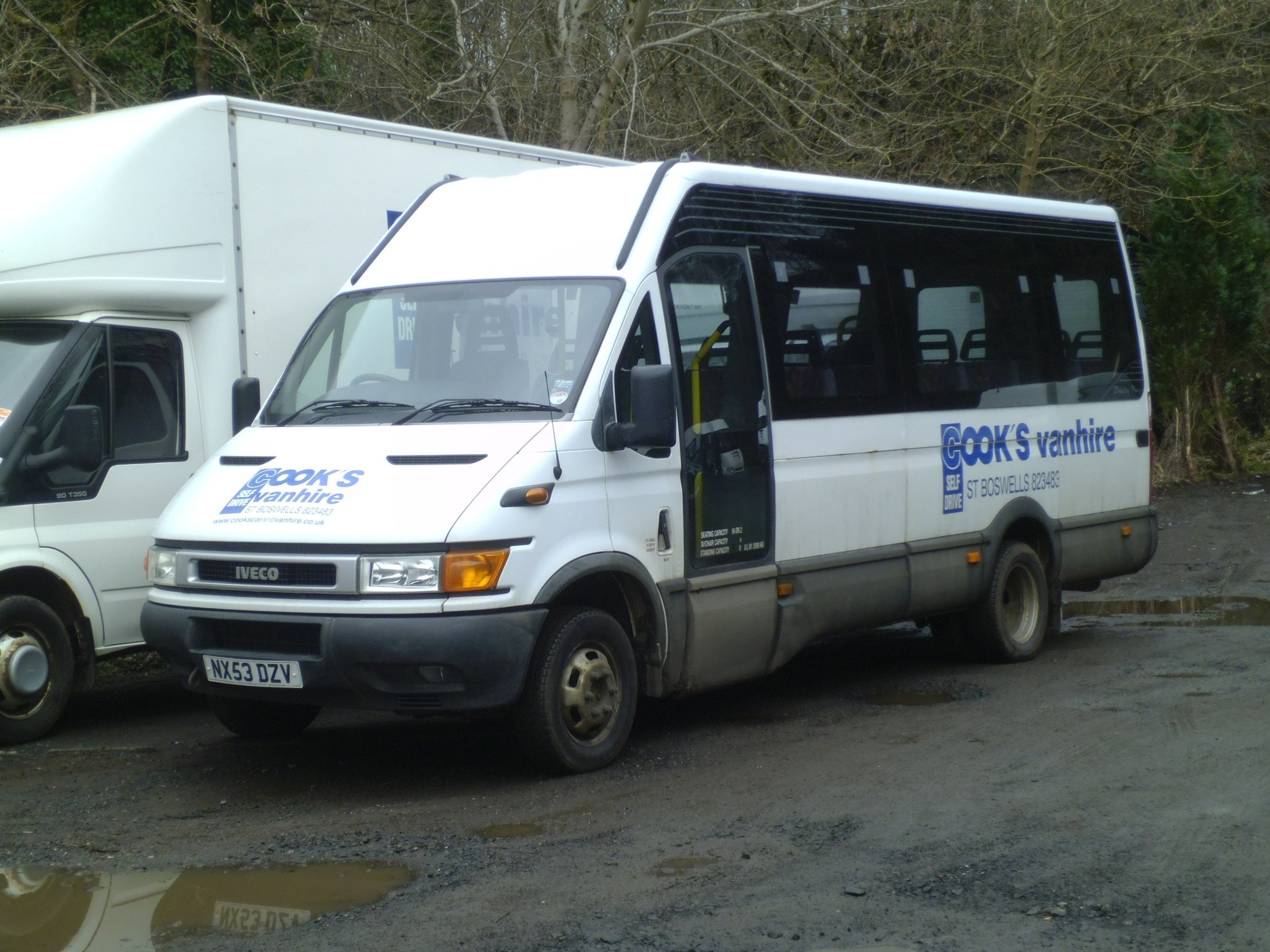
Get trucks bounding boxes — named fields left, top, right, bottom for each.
left=0, top=93, right=653, bottom=760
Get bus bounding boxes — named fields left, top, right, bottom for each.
left=131, top=152, right=1160, bottom=776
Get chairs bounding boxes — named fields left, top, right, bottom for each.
left=445, top=303, right=1106, bottom=478
